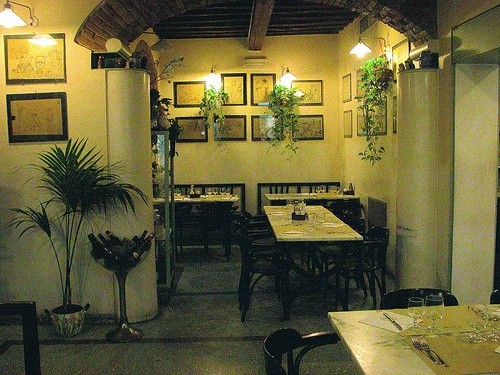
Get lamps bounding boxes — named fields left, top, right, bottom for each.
left=0, top=0, right=39, bottom=28
left=280, top=67, right=296, bottom=81
left=143, top=31, right=172, bottom=51
left=348, top=36, right=386, bottom=58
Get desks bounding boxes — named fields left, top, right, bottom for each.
left=327, top=304, right=500, bottom=375
left=263, top=206, right=363, bottom=320
left=265, top=192, right=360, bottom=226
left=153, top=193, right=238, bottom=261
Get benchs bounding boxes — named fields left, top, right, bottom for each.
left=168, top=183, right=245, bottom=246
left=257, top=182, right=341, bottom=214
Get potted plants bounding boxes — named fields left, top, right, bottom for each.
left=267, top=84, right=300, bottom=154
left=354, top=57, right=390, bottom=166
left=199, top=85, right=231, bottom=147
left=9, top=137, right=150, bottom=337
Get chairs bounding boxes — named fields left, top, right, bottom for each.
left=263, top=327, right=338, bottom=375
left=370, top=288, right=458, bottom=310
left=169, top=199, right=390, bottom=322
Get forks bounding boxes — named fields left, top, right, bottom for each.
left=419, top=339, right=449, bottom=367
left=412, top=338, right=441, bottom=365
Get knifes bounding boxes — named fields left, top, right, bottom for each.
left=383, top=312, right=402, bottom=330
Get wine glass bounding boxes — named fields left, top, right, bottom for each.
left=316, top=186, right=326, bottom=196
left=407, top=297, right=424, bottom=330
left=486, top=305, right=500, bottom=342
left=467, top=304, right=488, bottom=343
left=89, top=242, right=152, bottom=343
left=207, top=187, right=231, bottom=198
left=424, top=295, right=445, bottom=332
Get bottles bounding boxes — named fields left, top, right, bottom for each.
left=89, top=231, right=156, bottom=267
left=349, top=183, right=353, bottom=191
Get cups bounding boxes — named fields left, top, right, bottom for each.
left=305, top=212, right=325, bottom=227
left=329, top=186, right=342, bottom=196
left=176, top=190, right=181, bottom=196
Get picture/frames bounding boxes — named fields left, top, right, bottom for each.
left=292, top=115, right=324, bottom=141
left=291, top=80, right=324, bottom=106
left=173, top=82, right=207, bottom=108
left=175, top=116, right=208, bottom=143
left=4, top=33, right=67, bottom=85
left=214, top=73, right=283, bottom=141
left=342, top=38, right=411, bottom=138
left=6, top=91, right=68, bottom=143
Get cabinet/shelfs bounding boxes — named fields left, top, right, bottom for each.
left=151, top=130, right=176, bottom=290
